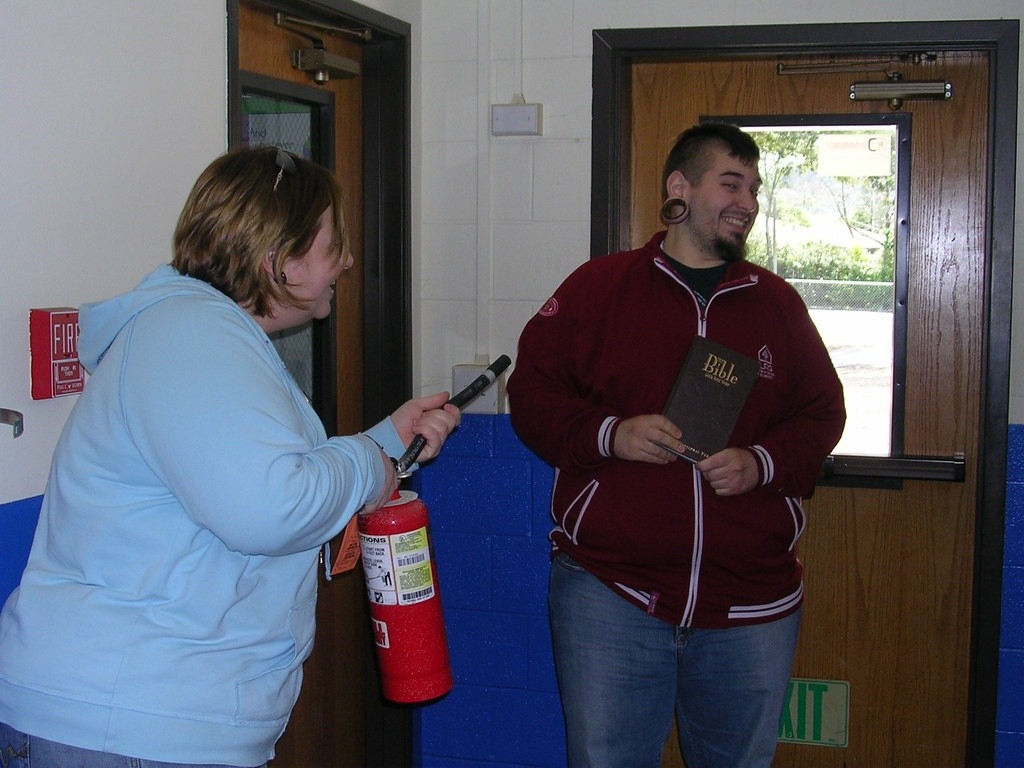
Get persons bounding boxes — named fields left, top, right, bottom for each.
left=506, top=123, right=847, bottom=767
left=1, top=147, right=461, bottom=768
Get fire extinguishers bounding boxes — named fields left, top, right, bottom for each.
left=352, top=352, right=517, bottom=708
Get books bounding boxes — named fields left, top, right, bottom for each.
left=649, top=335, right=761, bottom=473
